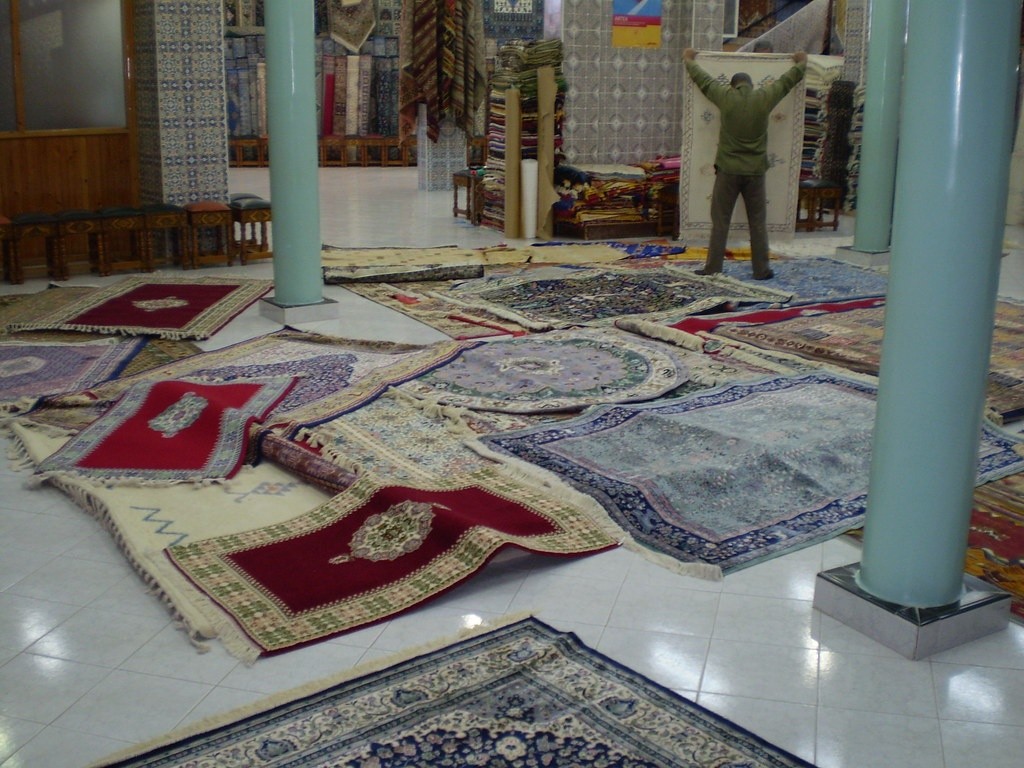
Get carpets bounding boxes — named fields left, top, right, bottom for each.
left=473, top=37, right=867, bottom=235
left=0, top=240, right=1024, bottom=768
left=221, top=0, right=490, bottom=143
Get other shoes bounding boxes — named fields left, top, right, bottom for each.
left=694, top=269, right=711, bottom=276
left=756, top=270, right=774, bottom=280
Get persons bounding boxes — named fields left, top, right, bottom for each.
left=685, top=48, right=808, bottom=279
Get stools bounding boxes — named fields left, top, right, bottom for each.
left=451, top=169, right=472, bottom=219
left=796, top=179, right=841, bottom=232
left=229, top=133, right=489, bottom=168
left=0, top=192, right=272, bottom=286
